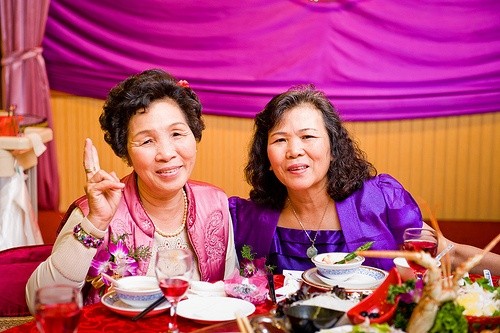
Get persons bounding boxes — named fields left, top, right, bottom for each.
left=229, top=84, right=500, bottom=275
left=25, top=69, right=240, bottom=322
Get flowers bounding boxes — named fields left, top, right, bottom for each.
left=85, top=226, right=153, bottom=298
left=352, top=272, right=467, bottom=333
left=226, top=245, right=277, bottom=304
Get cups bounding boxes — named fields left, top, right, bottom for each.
left=34, top=284, right=84, bottom=333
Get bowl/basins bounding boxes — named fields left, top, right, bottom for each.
left=113, top=276, right=163, bottom=308
left=347, top=257, right=441, bottom=327
left=311, top=252, right=365, bottom=282
left=459, top=273, right=500, bottom=333
left=285, top=305, right=345, bottom=333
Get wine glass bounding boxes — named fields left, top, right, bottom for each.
left=155, top=248, right=194, bottom=333
left=402, top=227, right=439, bottom=305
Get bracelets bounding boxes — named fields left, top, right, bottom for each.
left=73, top=223, right=104, bottom=249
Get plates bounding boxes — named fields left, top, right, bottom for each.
left=174, top=296, right=256, bottom=321
left=101, top=290, right=171, bottom=318
left=301, top=267, right=388, bottom=293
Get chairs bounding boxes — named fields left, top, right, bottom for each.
left=0, top=244, right=54, bottom=317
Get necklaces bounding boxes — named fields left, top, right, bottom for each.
left=287, top=194, right=334, bottom=259
left=139, top=188, right=188, bottom=238
